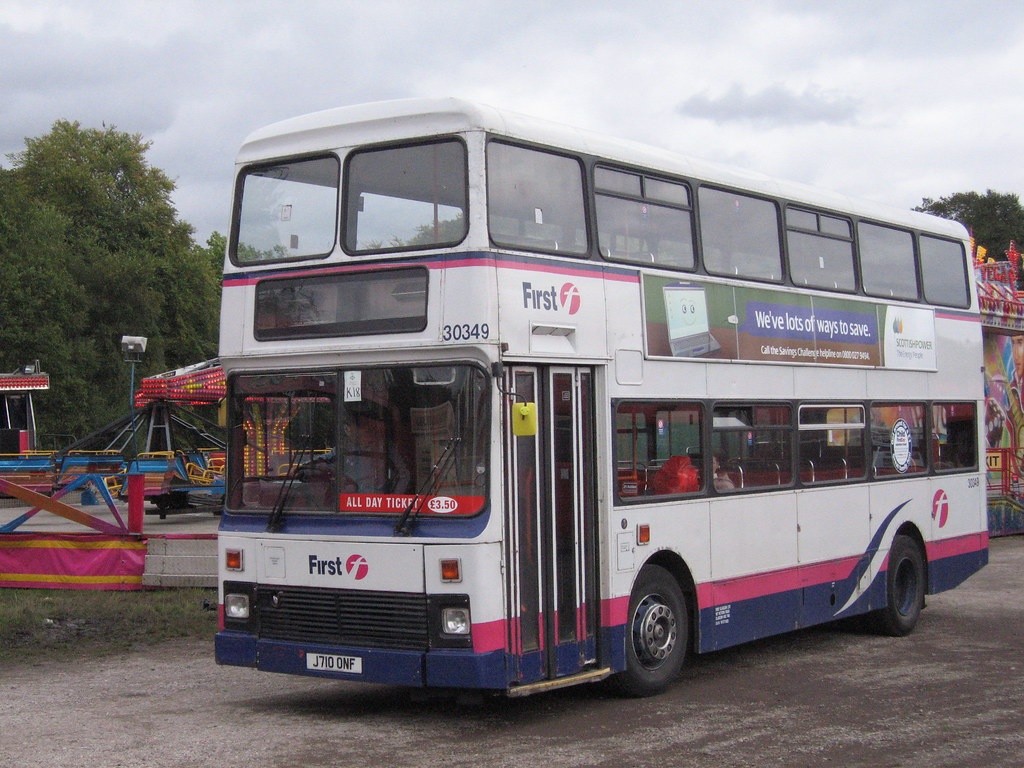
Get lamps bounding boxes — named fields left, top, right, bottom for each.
left=20, top=364, right=35, bottom=374
left=121, top=335, right=148, bottom=363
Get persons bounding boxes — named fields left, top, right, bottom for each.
left=293, top=408, right=411, bottom=495
left=711, top=453, right=740, bottom=490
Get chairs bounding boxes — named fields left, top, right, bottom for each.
left=617, top=432, right=940, bottom=519
left=546, top=240, right=893, bottom=297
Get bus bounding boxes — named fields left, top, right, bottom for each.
left=214, top=94, right=989, bottom=697
